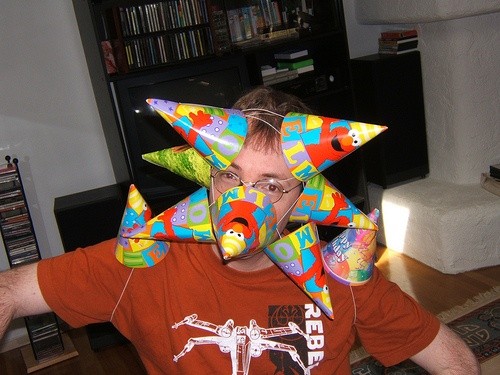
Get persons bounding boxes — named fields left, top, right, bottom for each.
left=0, top=86, right=481, bottom=375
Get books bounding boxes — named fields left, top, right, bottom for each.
left=0, top=166, right=59, bottom=344
left=119, top=0, right=215, bottom=71
left=260, top=49, right=315, bottom=87
left=226, top=0, right=289, bottom=42
left=378, top=29, right=419, bottom=55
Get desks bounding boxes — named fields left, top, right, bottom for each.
left=49, top=152, right=369, bottom=353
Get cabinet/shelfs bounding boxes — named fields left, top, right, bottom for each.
left=72, top=0, right=349, bottom=186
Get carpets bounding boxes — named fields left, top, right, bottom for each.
left=347, top=284, right=500, bottom=375
